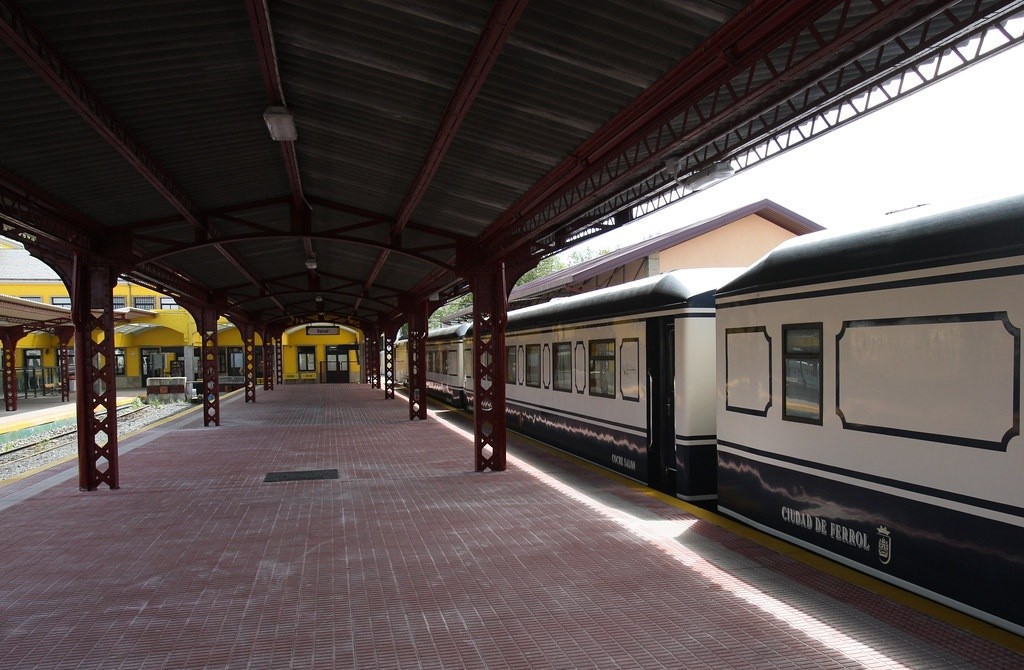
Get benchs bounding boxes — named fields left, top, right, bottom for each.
left=44, top=383, right=61, bottom=396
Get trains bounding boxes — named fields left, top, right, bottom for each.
left=396, top=193, right=1023, bottom=636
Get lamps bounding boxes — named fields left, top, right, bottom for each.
left=315, top=296, right=322, bottom=301
left=263, top=106, right=297, bottom=142
left=665, top=157, right=734, bottom=192
left=304, top=258, right=317, bottom=268
